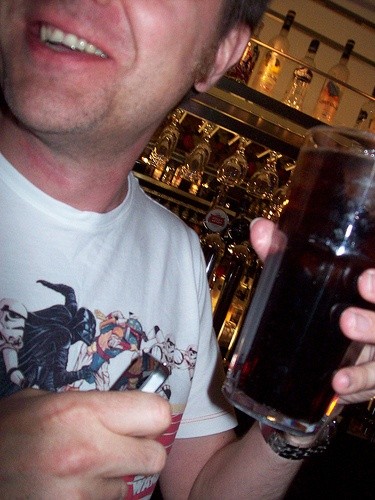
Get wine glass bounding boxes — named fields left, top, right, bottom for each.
left=248, top=149, right=280, bottom=199
left=267, top=165, right=295, bottom=215
left=180, top=123, right=215, bottom=183
left=217, top=135, right=250, bottom=187
left=144, top=108, right=185, bottom=170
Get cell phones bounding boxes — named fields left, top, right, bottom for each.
left=110, top=353, right=168, bottom=393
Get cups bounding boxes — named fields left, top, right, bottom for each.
left=220, top=123, right=375, bottom=438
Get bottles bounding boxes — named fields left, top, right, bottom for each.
left=201, top=213, right=260, bottom=354
left=224, top=17, right=263, bottom=88
left=283, top=35, right=320, bottom=112
left=354, top=84, right=375, bottom=132
left=312, top=39, right=355, bottom=125
left=249, top=9, right=296, bottom=97
